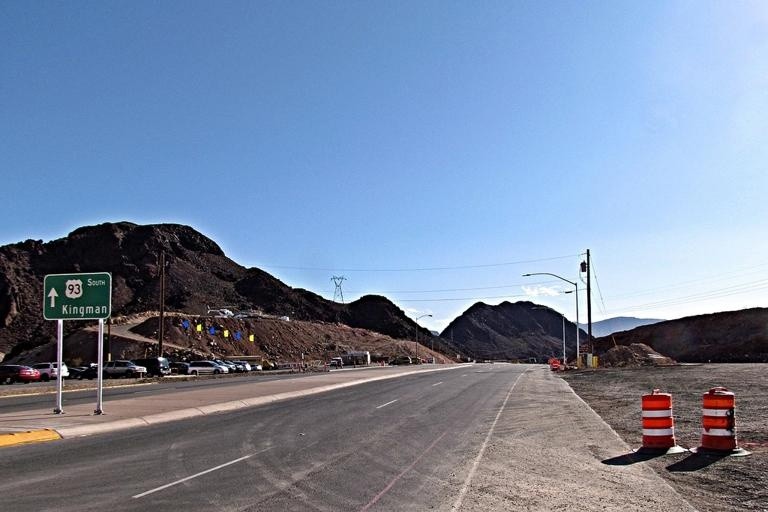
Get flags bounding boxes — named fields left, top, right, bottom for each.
left=183, top=320, right=254, bottom=343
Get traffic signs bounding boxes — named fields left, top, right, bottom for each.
left=44, top=271, right=111, bottom=321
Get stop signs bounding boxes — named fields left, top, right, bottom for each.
left=551, top=360, right=560, bottom=368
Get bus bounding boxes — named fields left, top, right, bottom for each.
left=225, top=355, right=275, bottom=370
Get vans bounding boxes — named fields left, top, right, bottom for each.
left=131, top=357, right=172, bottom=378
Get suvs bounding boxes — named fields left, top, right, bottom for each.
left=331, top=356, right=344, bottom=366
left=31, top=362, right=70, bottom=381
left=103, top=359, right=147, bottom=378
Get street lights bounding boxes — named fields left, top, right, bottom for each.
left=415, top=314, right=433, bottom=363
left=521, top=272, right=581, bottom=368
left=532, top=307, right=566, bottom=365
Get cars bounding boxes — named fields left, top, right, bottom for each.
left=169, top=359, right=263, bottom=375
left=67, top=361, right=98, bottom=380
left=389, top=354, right=428, bottom=365
left=0, top=364, right=40, bottom=385
left=209, top=307, right=265, bottom=320
